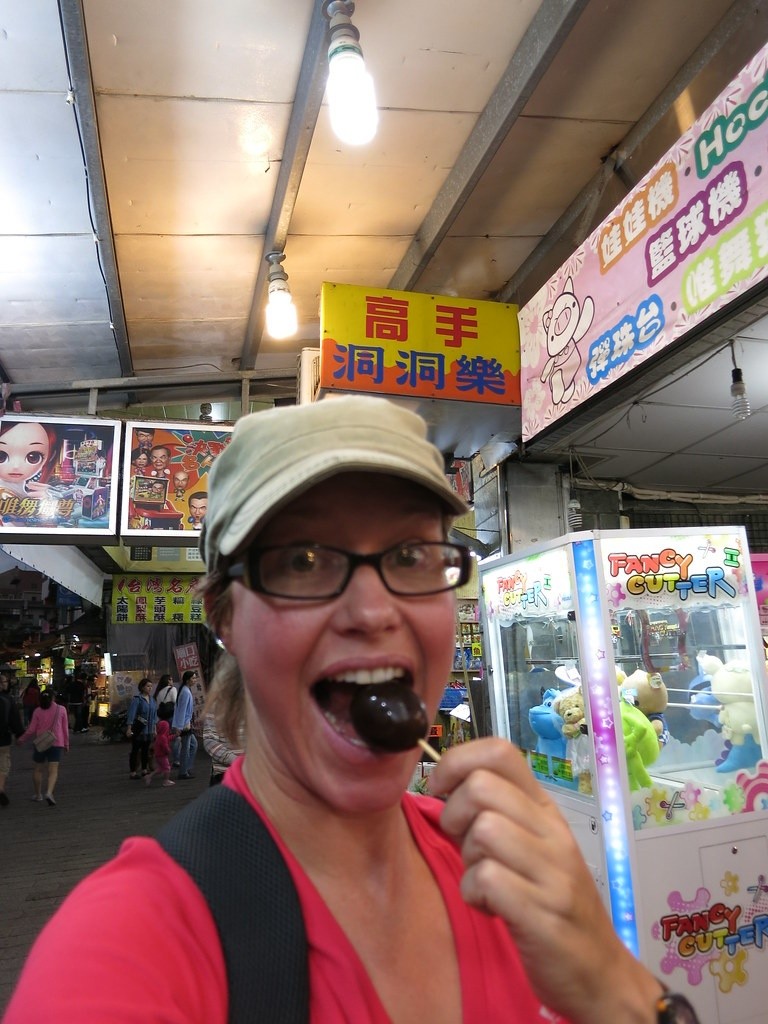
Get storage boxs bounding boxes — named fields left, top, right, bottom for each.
left=439, top=688, right=468, bottom=708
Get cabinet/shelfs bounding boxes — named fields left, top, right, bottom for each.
left=450, top=621, right=480, bottom=672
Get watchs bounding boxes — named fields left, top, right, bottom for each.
left=653, top=973, right=702, bottom=1024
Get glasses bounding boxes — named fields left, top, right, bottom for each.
left=221, top=540, right=473, bottom=601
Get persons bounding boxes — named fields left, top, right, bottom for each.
left=153, top=674, right=178, bottom=730
left=0, top=673, right=26, bottom=807
left=128, top=678, right=157, bottom=780
left=8, top=672, right=20, bottom=694
left=15, top=687, right=70, bottom=806
left=23, top=677, right=41, bottom=725
left=0, top=392, right=707, bottom=1024
left=172, top=671, right=199, bottom=780
left=143, top=719, right=180, bottom=789
left=69, top=673, right=99, bottom=733
left=55, top=686, right=72, bottom=729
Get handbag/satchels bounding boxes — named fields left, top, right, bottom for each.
left=156, top=701, right=177, bottom=720
left=32, top=729, right=56, bottom=753
left=128, top=716, right=147, bottom=740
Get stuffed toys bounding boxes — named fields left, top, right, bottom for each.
left=529, top=650, right=763, bottom=795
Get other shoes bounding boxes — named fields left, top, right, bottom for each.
left=176, top=772, right=196, bottom=779
left=162, top=781, right=175, bottom=786
left=0, top=791, right=10, bottom=806
left=129, top=774, right=142, bottom=780
left=44, top=792, right=57, bottom=806
left=30, top=793, right=42, bottom=802
left=172, top=762, right=181, bottom=768
left=144, top=774, right=152, bottom=787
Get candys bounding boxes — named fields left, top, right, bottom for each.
left=349, top=680, right=427, bottom=752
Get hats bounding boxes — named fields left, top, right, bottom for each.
left=196, top=393, right=471, bottom=582
